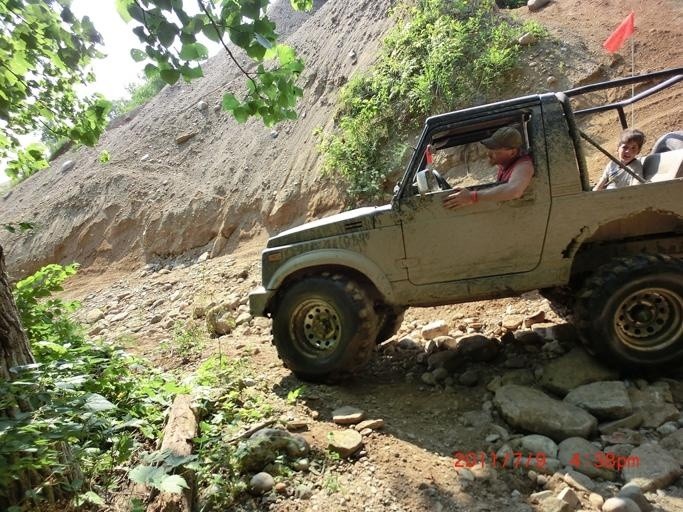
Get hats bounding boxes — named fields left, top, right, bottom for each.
left=480, top=126, right=523, bottom=150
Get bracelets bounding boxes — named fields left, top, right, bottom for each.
left=471, top=190, right=477, bottom=203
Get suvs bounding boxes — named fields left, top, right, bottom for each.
left=247, top=69, right=683, bottom=380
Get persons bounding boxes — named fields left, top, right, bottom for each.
left=441, top=126, right=532, bottom=210
left=589, top=129, right=648, bottom=191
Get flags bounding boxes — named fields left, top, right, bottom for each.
left=601, top=12, right=635, bottom=54
